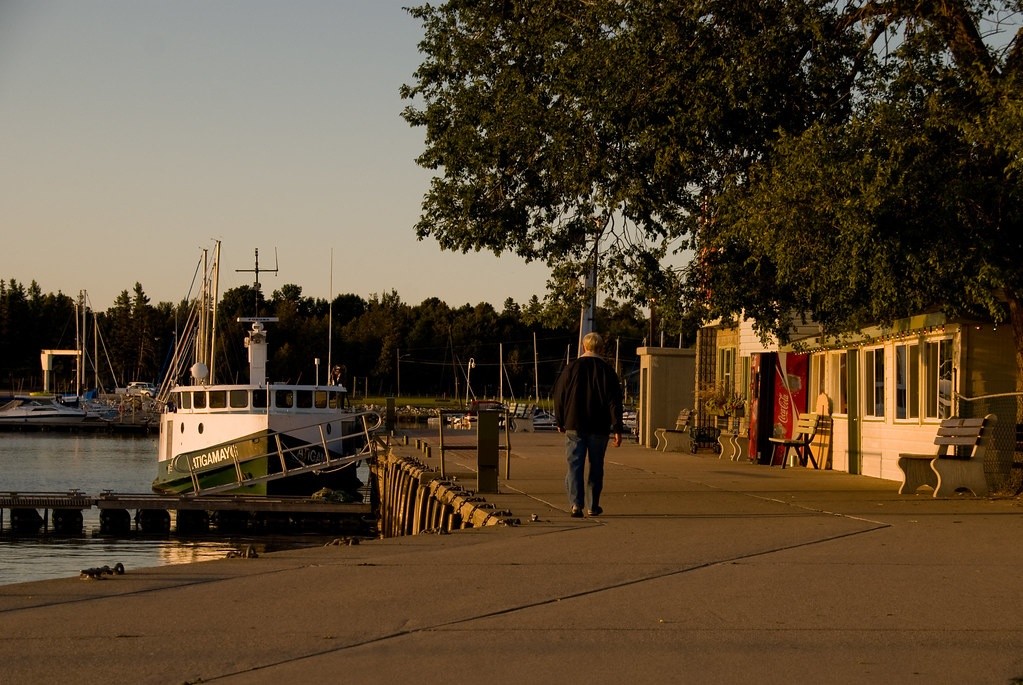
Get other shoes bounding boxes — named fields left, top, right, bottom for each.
left=588, top=506, right=602, bottom=515
left=571, top=510, right=583, bottom=517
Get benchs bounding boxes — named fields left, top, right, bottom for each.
left=653, top=408, right=693, bottom=452
left=767, top=412, right=820, bottom=471
left=893, top=413, right=998, bottom=497
left=501, top=403, right=536, bottom=433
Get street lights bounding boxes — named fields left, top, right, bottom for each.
left=465, top=357, right=475, bottom=405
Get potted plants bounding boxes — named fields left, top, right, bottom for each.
left=686, top=379, right=747, bottom=418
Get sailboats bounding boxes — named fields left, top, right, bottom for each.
left=0, top=237, right=360, bottom=499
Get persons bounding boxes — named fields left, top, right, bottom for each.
left=553, top=332, right=623, bottom=516
